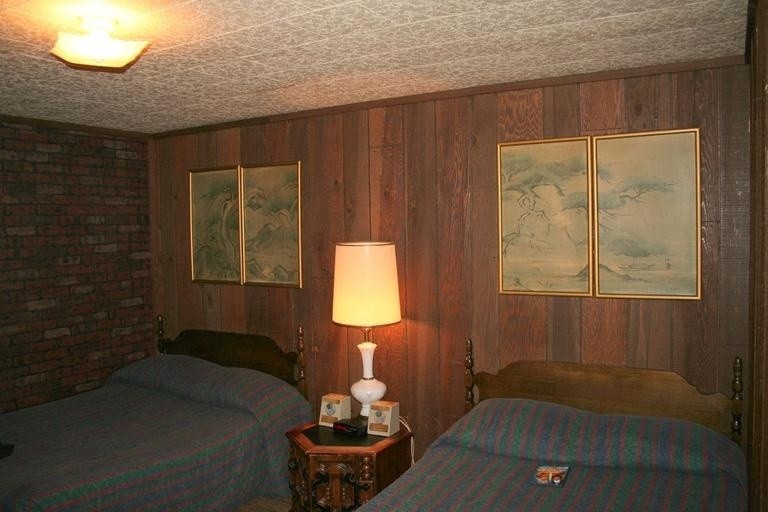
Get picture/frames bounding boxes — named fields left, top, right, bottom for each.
left=187, top=159, right=303, bottom=291
left=494, top=126, right=702, bottom=302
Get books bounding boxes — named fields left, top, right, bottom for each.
left=527, top=464, right=570, bottom=490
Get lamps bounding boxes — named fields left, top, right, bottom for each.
left=331, top=241, right=404, bottom=419
